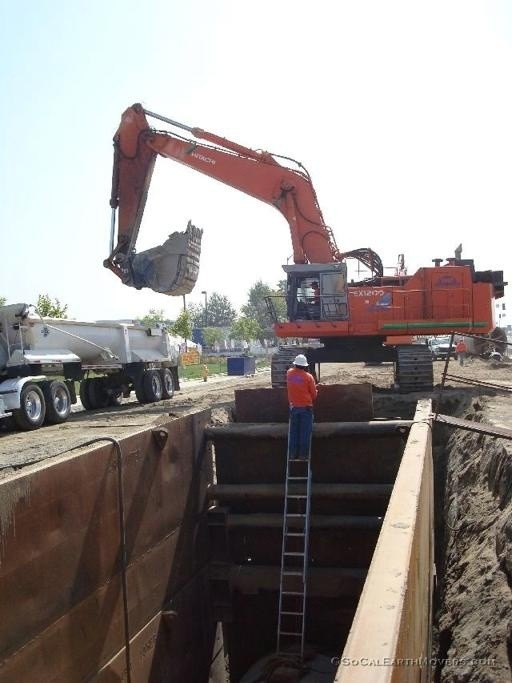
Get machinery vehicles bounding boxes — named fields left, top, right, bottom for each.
left=105, top=100, right=507, bottom=394
left=1, top=299, right=181, bottom=429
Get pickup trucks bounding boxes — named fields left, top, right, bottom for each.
left=419, top=335, right=461, bottom=362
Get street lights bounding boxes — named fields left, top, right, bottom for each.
left=201, top=290, right=209, bottom=328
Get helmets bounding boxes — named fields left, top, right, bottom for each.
left=293, top=354, right=308, bottom=366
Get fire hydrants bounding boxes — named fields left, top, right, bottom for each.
left=201, top=364, right=209, bottom=383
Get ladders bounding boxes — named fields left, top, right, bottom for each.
left=276, top=410, right=314, bottom=662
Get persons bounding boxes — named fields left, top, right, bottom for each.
left=307, top=280, right=320, bottom=317
left=455, top=338, right=467, bottom=366
left=286, top=352, right=318, bottom=460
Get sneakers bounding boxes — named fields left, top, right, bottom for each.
left=290, top=456, right=308, bottom=459
